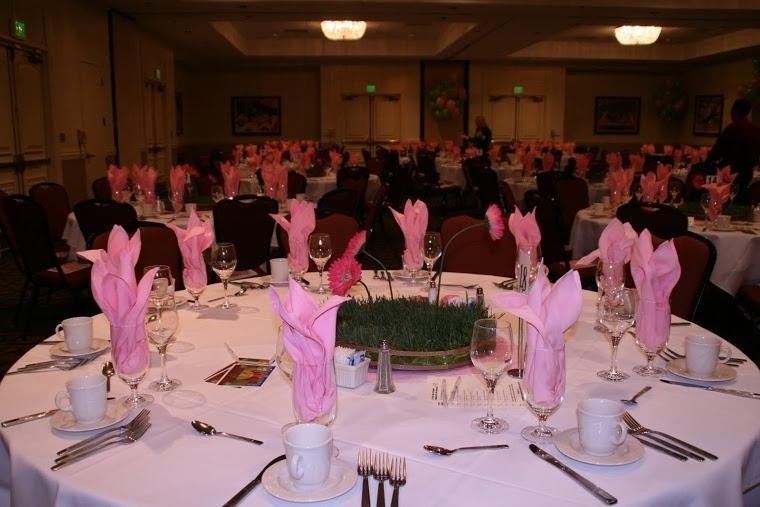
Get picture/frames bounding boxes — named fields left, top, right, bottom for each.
left=595, top=95, right=643, bottom=135
left=692, top=93, right=723, bottom=137
left=230, top=94, right=282, bottom=137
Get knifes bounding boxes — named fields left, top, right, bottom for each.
left=528, top=443, right=619, bottom=505
left=657, top=377, right=760, bottom=406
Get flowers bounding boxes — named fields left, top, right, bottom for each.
left=327, top=202, right=506, bottom=367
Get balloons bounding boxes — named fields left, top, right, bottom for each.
left=428, top=79, right=467, bottom=120
left=649, top=79, right=690, bottom=120
left=736, top=79, right=760, bottom=100
left=751, top=55, right=760, bottom=78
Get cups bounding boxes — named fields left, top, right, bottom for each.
left=270, top=257, right=290, bottom=282
left=683, top=332, right=732, bottom=378
left=54, top=316, right=94, bottom=353
left=281, top=421, right=334, bottom=491
left=574, top=398, right=627, bottom=457
left=54, top=373, right=109, bottom=427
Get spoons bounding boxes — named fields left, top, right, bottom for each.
left=190, top=419, right=265, bottom=448
left=621, top=385, right=652, bottom=406
left=422, top=443, right=511, bottom=459
left=6, top=354, right=102, bottom=375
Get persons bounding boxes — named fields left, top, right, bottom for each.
left=713, top=98, right=759, bottom=192
left=460, top=114, right=493, bottom=158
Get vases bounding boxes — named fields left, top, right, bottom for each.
left=334, top=338, right=496, bottom=370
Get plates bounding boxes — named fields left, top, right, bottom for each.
left=261, top=457, right=358, bottom=503
left=50, top=338, right=109, bottom=357
left=665, top=356, right=739, bottom=382
left=552, top=428, right=646, bottom=466
left=48, top=402, right=128, bottom=434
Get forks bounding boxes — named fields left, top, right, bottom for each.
left=624, top=411, right=721, bottom=463
left=356, top=445, right=409, bottom=507
left=49, top=409, right=156, bottom=475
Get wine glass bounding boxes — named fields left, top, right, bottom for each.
left=289, top=232, right=333, bottom=295
left=276, top=321, right=340, bottom=458
left=606, top=185, right=760, bottom=229
left=470, top=318, right=569, bottom=445
left=401, top=230, right=443, bottom=293
left=514, top=242, right=542, bottom=293
left=144, top=242, right=237, bottom=318
left=109, top=182, right=291, bottom=217
left=109, top=295, right=181, bottom=409
left=593, top=258, right=671, bottom=383
